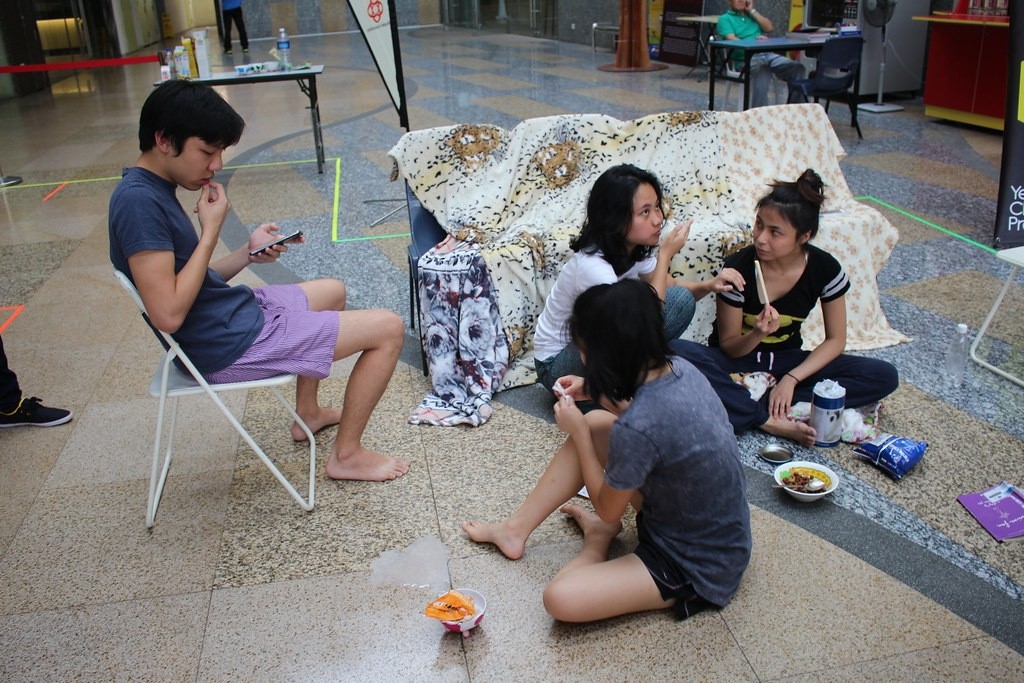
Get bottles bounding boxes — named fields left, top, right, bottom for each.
left=277, top=27, right=292, bottom=72
left=945, top=323, right=968, bottom=388
left=165, top=47, right=174, bottom=67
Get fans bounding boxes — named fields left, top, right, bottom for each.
left=856, top=0, right=906, bottom=113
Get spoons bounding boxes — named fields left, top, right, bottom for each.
left=771, top=480, right=826, bottom=491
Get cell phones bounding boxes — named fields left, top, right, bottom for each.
left=249, top=231, right=304, bottom=255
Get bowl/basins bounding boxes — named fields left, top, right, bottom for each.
left=774, top=461, right=840, bottom=502
left=440, top=588, right=487, bottom=632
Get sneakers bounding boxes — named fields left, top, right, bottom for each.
left=0, top=397, right=73, bottom=428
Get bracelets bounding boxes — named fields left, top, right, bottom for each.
left=786, top=371, right=799, bottom=383
left=750, top=8, right=758, bottom=15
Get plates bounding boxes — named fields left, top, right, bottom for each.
left=758, top=444, right=794, bottom=463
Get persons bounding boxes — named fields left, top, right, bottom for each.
left=532, top=165, right=746, bottom=414
left=0, top=334, right=73, bottom=427
left=463, top=278, right=752, bottom=622
left=668, top=168, right=898, bottom=448
left=714, top=0, right=805, bottom=108
left=221, top=0, right=249, bottom=56
left=108, top=80, right=412, bottom=481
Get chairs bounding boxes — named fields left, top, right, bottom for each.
left=111, top=264, right=316, bottom=529
left=405, top=178, right=448, bottom=377
left=787, top=36, right=864, bottom=138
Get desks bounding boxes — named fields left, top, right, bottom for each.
left=708, top=36, right=866, bottom=127
left=676, top=15, right=727, bottom=82
left=152, top=64, right=325, bottom=174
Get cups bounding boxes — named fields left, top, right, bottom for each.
left=810, top=389, right=847, bottom=447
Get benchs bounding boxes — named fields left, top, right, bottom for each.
left=591, top=21, right=649, bottom=53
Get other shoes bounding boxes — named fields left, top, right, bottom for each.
left=243, top=48, right=248, bottom=52
left=224, top=49, right=232, bottom=54
